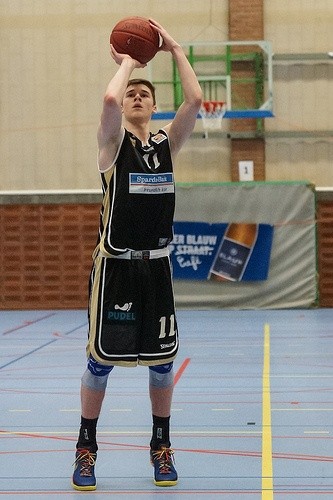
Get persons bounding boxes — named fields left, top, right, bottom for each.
left=67, top=18, right=205, bottom=492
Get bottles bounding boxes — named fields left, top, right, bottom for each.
left=207, top=221, right=260, bottom=282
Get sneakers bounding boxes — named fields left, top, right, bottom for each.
left=149, top=441, right=178, bottom=486
left=72, top=443, right=100, bottom=491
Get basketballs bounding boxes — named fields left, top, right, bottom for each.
left=110, top=16, right=160, bottom=65
